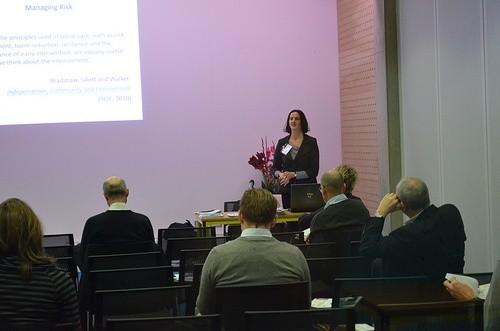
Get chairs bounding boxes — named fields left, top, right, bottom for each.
left=40, top=202, right=493, bottom=331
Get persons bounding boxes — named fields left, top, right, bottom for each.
left=298, top=163, right=370, bottom=234
left=359, top=177, right=468, bottom=280
left=0, top=198, right=81, bottom=331
left=271, top=109, right=321, bottom=210
left=306, top=169, right=370, bottom=244
left=194, top=187, right=312, bottom=315
left=443, top=259, right=500, bottom=331
left=77, top=175, right=156, bottom=305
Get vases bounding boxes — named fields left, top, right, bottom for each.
left=273, top=194, right=284, bottom=211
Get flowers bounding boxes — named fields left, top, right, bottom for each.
left=248, top=136, right=281, bottom=193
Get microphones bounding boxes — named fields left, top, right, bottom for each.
left=249, top=180, right=254, bottom=190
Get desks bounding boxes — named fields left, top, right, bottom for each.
left=194, top=208, right=316, bottom=238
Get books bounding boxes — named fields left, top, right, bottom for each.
left=193, top=209, right=224, bottom=217
left=445, top=272, right=488, bottom=300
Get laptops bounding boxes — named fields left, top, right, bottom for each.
left=288, top=183, right=326, bottom=212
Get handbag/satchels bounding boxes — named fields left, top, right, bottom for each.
left=164, top=220, right=194, bottom=240
